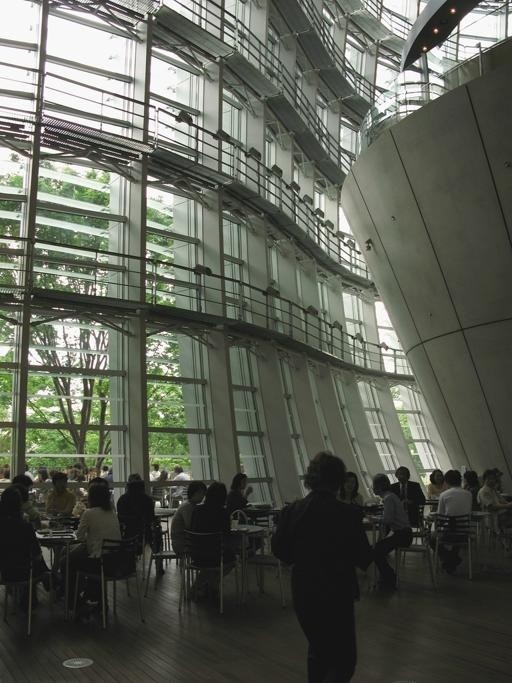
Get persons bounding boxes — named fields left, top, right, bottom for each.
left=68, top=472, right=119, bottom=616
left=115, top=462, right=192, bottom=576
left=340, top=466, right=427, bottom=593
left=0, top=475, right=62, bottom=606
left=272, top=449, right=368, bottom=682
left=429, top=467, right=510, bottom=583
left=3, top=464, right=112, bottom=575
left=170, top=467, right=265, bottom=605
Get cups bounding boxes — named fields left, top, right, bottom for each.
left=85, top=474, right=90, bottom=481
left=232, top=519, right=239, bottom=528
left=41, top=520, right=50, bottom=534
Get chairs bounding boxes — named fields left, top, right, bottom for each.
left=361, top=488, right=511, bottom=592
left=58, top=467, right=192, bottom=507
left=0, top=490, right=289, bottom=628
